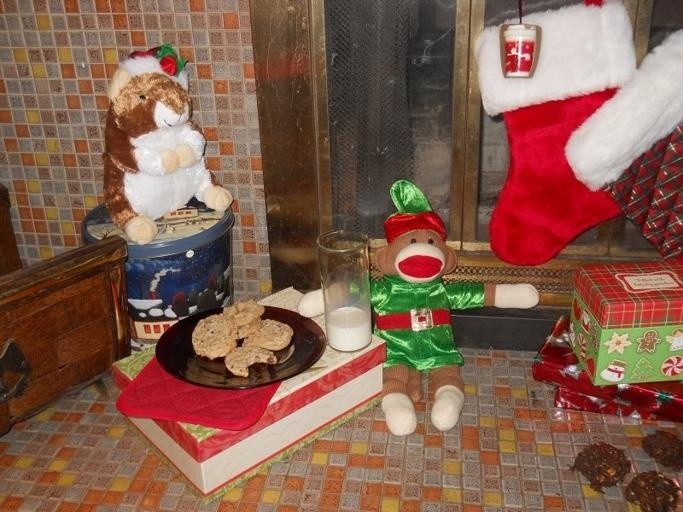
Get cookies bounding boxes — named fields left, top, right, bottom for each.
left=566, top=441, right=632, bottom=494
left=624, top=470, right=680, bottom=512
left=191, top=298, right=296, bottom=378
left=642, top=430, right=683, bottom=472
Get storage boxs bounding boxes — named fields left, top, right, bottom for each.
left=110, top=286, right=386, bottom=504
left=569, top=262, right=683, bottom=386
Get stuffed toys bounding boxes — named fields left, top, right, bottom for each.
left=296, top=178, right=541, bottom=438
left=102, top=44, right=234, bottom=246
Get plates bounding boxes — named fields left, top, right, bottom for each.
left=154, top=304, right=325, bottom=387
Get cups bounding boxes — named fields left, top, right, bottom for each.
left=501, top=25, right=537, bottom=78
left=317, top=227, right=374, bottom=354
